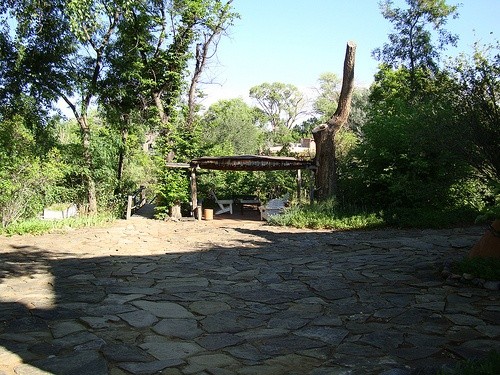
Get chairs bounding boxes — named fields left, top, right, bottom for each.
left=211, top=189, right=233, bottom=215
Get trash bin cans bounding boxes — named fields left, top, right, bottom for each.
left=204, top=208, right=215, bottom=221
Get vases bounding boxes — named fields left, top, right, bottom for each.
left=204, top=209, right=213, bottom=221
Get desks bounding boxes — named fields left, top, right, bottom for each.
left=240, top=199, right=261, bottom=215
